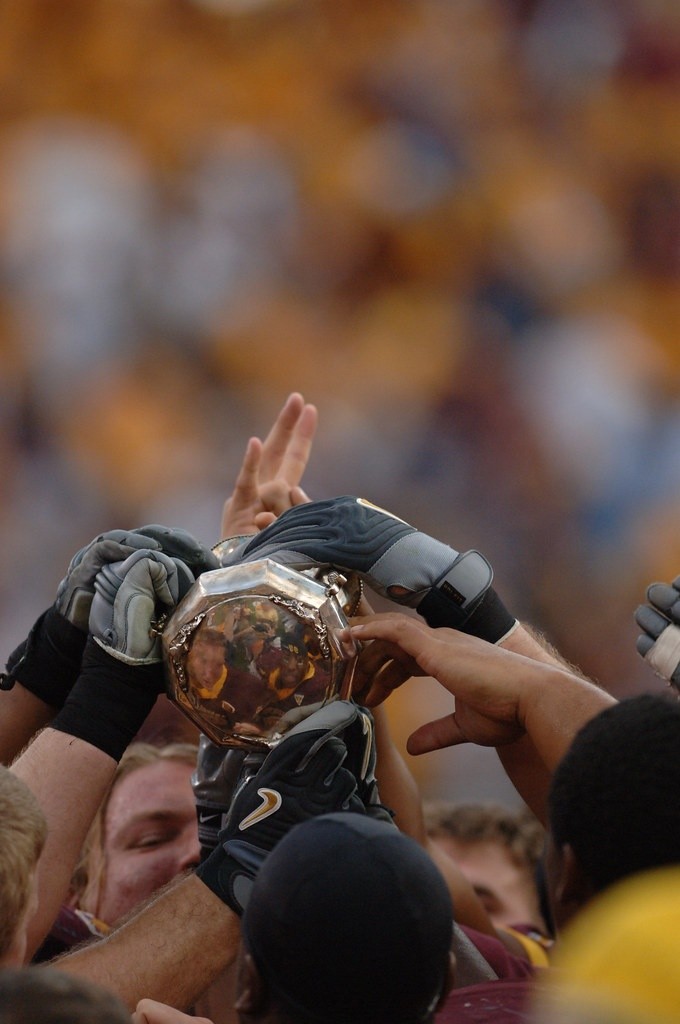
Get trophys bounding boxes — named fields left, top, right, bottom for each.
left=150, top=535, right=364, bottom=752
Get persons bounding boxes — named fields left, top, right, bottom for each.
left=0, top=391, right=680, bottom=1024
left=231, top=809, right=456, bottom=1024
left=71, top=739, right=240, bottom=1024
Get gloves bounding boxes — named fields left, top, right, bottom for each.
left=196, top=699, right=374, bottom=918
left=49, top=548, right=195, bottom=758
left=12, top=525, right=219, bottom=700
left=244, top=490, right=494, bottom=627
left=633, top=576, right=680, bottom=690
left=195, top=748, right=270, bottom=863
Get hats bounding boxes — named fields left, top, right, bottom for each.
left=549, top=693, right=680, bottom=874
left=243, top=811, right=452, bottom=1024
